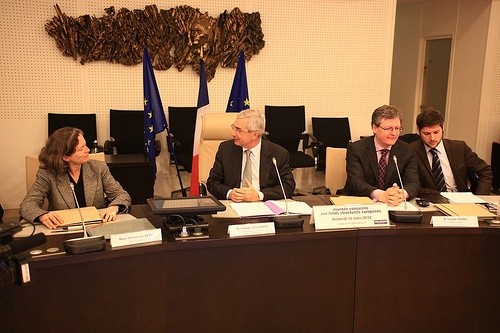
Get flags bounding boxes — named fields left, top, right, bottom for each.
left=226, top=53, right=250, bottom=112
left=143, top=48, right=166, bottom=161
left=189, top=60, right=210, bottom=196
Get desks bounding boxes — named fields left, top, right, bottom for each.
left=105, top=154, right=156, bottom=204
left=0, top=195, right=500, bottom=333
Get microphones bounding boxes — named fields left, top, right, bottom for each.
left=388, top=155, right=423, bottom=224
left=268, top=156, right=304, bottom=228
left=62, top=182, right=105, bottom=255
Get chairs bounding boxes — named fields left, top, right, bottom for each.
left=325, top=147, right=348, bottom=196
left=312, top=117, right=352, bottom=170
left=167, top=106, right=199, bottom=196
left=200, top=113, right=241, bottom=195
left=262, top=106, right=318, bottom=172
left=47, top=113, right=97, bottom=155
left=104, top=109, right=161, bottom=159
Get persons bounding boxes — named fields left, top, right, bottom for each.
left=346, top=105, right=421, bottom=207
left=18, top=127, right=132, bottom=229
left=410, top=111, right=493, bottom=192
left=207, top=109, right=296, bottom=203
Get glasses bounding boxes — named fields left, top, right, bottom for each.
left=231, top=124, right=258, bottom=133
left=378, top=125, right=402, bottom=133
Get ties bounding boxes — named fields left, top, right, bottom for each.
left=429, top=148, right=447, bottom=192
left=378, top=150, right=388, bottom=189
left=242, top=150, right=252, bottom=188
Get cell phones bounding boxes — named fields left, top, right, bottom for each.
left=416, top=200, right=429, bottom=207
